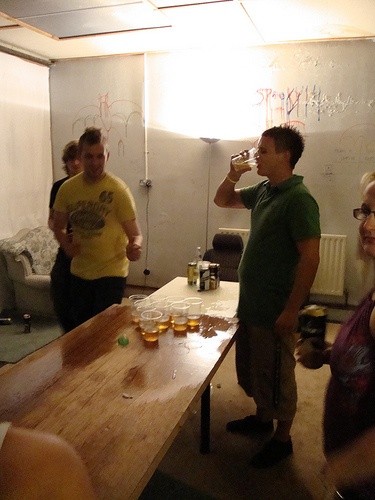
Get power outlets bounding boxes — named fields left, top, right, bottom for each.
left=140, top=178, right=152, bottom=189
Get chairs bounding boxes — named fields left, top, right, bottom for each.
left=202, top=233, right=244, bottom=282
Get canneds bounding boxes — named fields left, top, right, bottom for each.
left=299, top=305, right=328, bottom=369
left=24, top=314, right=31, bottom=332
left=0, top=318, right=12, bottom=324
left=187, top=262, right=198, bottom=284
left=200, top=263, right=220, bottom=291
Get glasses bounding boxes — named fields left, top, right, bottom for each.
left=353, top=208, right=375, bottom=220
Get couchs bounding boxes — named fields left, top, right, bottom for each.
left=0, top=226, right=60, bottom=322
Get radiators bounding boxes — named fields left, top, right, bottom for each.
left=219, top=228, right=347, bottom=296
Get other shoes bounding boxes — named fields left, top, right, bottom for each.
left=251, top=436, right=293, bottom=468
left=227, top=415, right=273, bottom=434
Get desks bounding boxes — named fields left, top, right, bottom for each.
left=0, top=276, right=241, bottom=500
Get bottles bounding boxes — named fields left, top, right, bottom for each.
left=192, top=247, right=202, bottom=285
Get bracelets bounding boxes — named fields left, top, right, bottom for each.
left=227, top=174, right=237, bottom=183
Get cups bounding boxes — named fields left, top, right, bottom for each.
left=153, top=301, right=173, bottom=330
left=149, top=294, right=168, bottom=305
left=231, top=147, right=261, bottom=173
left=129, top=294, right=148, bottom=322
left=140, top=310, right=163, bottom=342
left=168, top=296, right=186, bottom=323
left=134, top=300, right=158, bottom=318
left=184, top=297, right=203, bottom=326
left=171, top=302, right=190, bottom=331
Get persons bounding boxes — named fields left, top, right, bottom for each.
left=213, top=126, right=321, bottom=469
left=0, top=422, right=95, bottom=500
left=296, top=172, right=375, bottom=500
left=48, top=128, right=143, bottom=332
